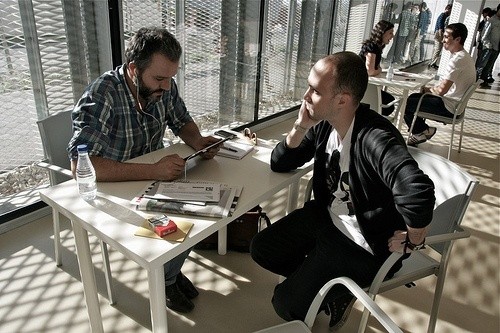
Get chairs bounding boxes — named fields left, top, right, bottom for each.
left=407, top=78, right=486, bottom=158
left=356, top=144, right=479, bottom=332
left=258, top=277, right=401, bottom=332
left=37, top=108, right=119, bottom=306
left=363, top=84, right=405, bottom=127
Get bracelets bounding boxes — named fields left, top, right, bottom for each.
left=293, top=121, right=310, bottom=133
left=400, top=231, right=426, bottom=257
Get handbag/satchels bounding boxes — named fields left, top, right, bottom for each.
left=194, top=205, right=272, bottom=253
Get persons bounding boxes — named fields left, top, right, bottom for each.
left=429, top=3, right=455, bottom=69
left=65, top=24, right=224, bottom=313
left=248, top=51, right=439, bottom=328
left=469, top=3, right=500, bottom=89
left=402, top=21, right=478, bottom=148
left=369, top=0, right=432, bottom=66
left=349, top=20, right=395, bottom=116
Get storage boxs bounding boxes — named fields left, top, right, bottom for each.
left=145, top=215, right=176, bottom=239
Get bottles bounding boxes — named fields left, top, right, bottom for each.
left=386, top=60, right=394, bottom=82
left=76, top=145, right=97, bottom=201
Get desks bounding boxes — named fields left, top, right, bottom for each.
left=367, top=71, right=434, bottom=133
left=40, top=129, right=316, bottom=333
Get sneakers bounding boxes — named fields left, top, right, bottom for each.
left=328, top=293, right=357, bottom=331
left=424, top=127, right=437, bottom=140
left=405, top=132, right=427, bottom=145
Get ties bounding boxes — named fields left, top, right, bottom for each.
left=326, top=150, right=341, bottom=195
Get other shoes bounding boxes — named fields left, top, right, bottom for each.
left=481, top=74, right=494, bottom=83
left=166, top=282, right=194, bottom=313
left=480, top=82, right=491, bottom=89
left=428, top=64, right=439, bottom=68
left=176, top=271, right=199, bottom=299
